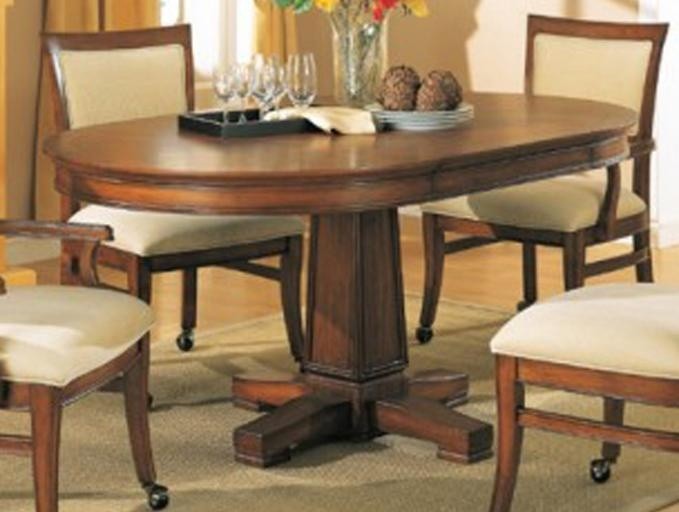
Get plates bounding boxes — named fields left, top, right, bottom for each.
left=367, top=101, right=475, bottom=135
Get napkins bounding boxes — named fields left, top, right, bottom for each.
left=266, top=106, right=382, bottom=136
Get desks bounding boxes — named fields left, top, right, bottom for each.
left=42, top=90, right=637, bottom=468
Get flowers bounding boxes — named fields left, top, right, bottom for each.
left=279, top=0, right=430, bottom=87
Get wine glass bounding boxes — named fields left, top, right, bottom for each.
left=211, top=52, right=318, bottom=123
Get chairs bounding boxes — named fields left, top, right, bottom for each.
left=415, top=13, right=670, bottom=345
left=38, top=22, right=306, bottom=401
left=490, top=280, right=678, bottom=511
left=1, top=219, right=170, bottom=511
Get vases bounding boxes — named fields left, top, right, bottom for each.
left=326, top=1, right=389, bottom=105
